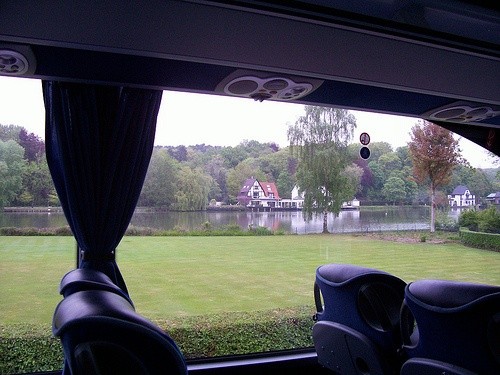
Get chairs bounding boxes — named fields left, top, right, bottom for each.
left=51, top=288, right=188, bottom=375
left=398, top=280, right=500, bottom=375
left=310, top=263, right=409, bottom=375
left=59, top=265, right=137, bottom=309
